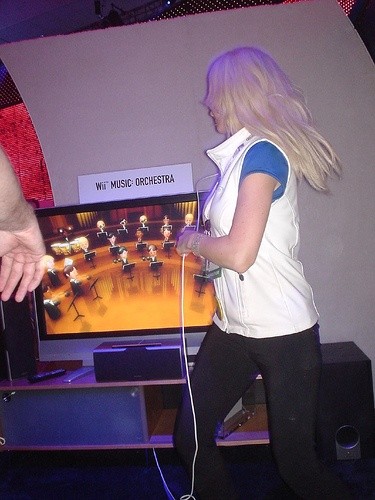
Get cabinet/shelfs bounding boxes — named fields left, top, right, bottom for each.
left=0, top=362, right=269, bottom=453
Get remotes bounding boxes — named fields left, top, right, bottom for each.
left=26, top=367, right=66, bottom=384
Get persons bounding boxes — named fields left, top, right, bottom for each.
left=63, top=265, right=81, bottom=296
left=120, top=218, right=127, bottom=229
left=163, top=227, right=172, bottom=243
left=139, top=215, right=147, bottom=227
left=42, top=285, right=61, bottom=321
left=43, top=255, right=60, bottom=285
left=185, top=213, right=193, bottom=226
left=0, top=147, right=46, bottom=303
left=147, top=244, right=158, bottom=263
left=117, top=247, right=128, bottom=268
left=79, top=237, right=89, bottom=253
left=162, top=214, right=170, bottom=226
left=107, top=230, right=117, bottom=247
left=135, top=229, right=144, bottom=243
left=97, top=220, right=105, bottom=232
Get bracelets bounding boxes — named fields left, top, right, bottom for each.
left=171, top=46, right=360, bottom=500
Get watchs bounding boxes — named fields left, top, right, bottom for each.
left=192, top=232, right=202, bottom=256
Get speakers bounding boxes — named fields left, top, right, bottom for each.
left=316, top=341, right=375, bottom=463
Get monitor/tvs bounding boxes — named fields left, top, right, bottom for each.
left=33, top=191, right=218, bottom=341
